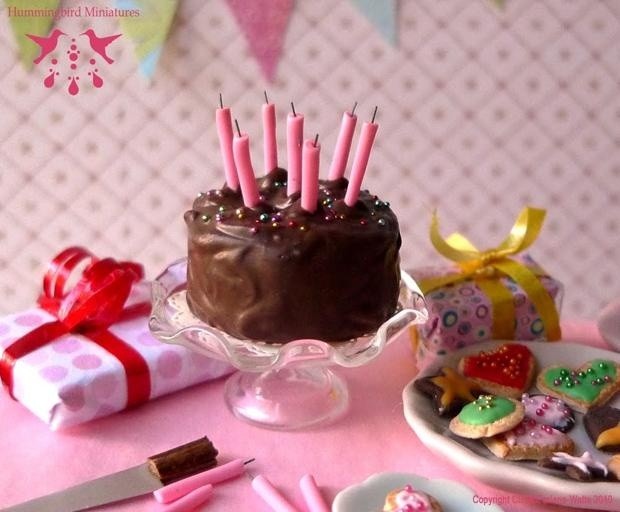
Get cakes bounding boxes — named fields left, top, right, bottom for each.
left=185, top=168, right=404, bottom=346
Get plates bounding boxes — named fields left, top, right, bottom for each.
left=404, top=339, right=620, bottom=508
left=332, top=469, right=503, bottom=512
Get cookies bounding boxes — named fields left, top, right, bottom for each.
left=383, top=343, right=620, bottom=512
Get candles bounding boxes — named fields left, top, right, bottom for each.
left=243, top=470, right=299, bottom=512
left=328, top=100, right=358, bottom=181
left=153, top=457, right=255, bottom=504
left=215, top=92, right=239, bottom=192
left=297, top=474, right=331, bottom=512
left=153, top=484, right=217, bottom=511
left=343, top=106, right=379, bottom=206
left=261, top=90, right=279, bottom=175
left=286, top=101, right=304, bottom=196
left=231, top=119, right=261, bottom=208
left=301, top=135, right=321, bottom=213
